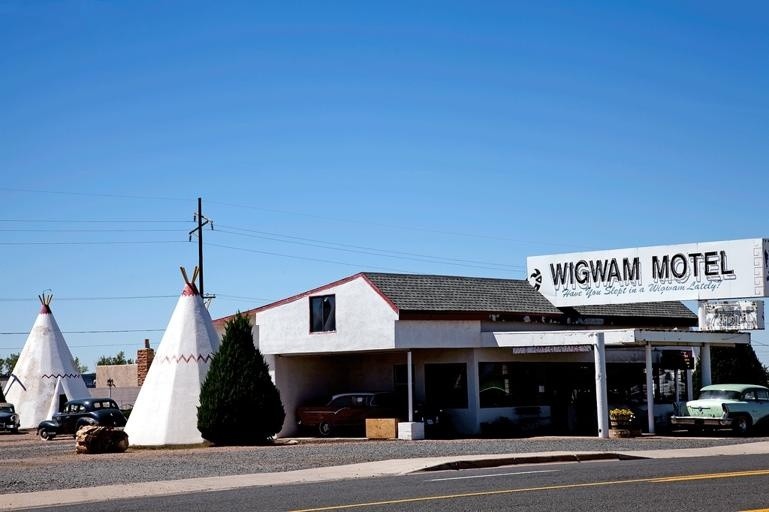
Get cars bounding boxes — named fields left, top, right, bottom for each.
left=0, top=402, right=20, bottom=434
left=670, top=383, right=769, bottom=436
left=36, top=398, right=132, bottom=441
left=295, top=392, right=449, bottom=437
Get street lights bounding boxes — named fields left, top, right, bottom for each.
left=107, top=378, right=113, bottom=397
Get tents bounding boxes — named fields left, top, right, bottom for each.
left=3, top=304, right=95, bottom=430
left=123, top=283, right=222, bottom=448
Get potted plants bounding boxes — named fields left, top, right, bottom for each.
left=609, top=408, right=635, bottom=428
